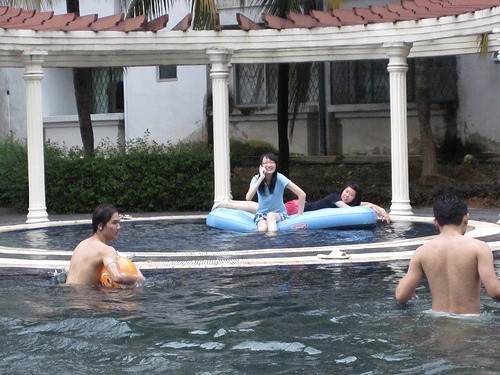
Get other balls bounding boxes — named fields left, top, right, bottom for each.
left=100, top=257, right=140, bottom=289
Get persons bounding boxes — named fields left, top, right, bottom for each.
left=395, top=195, right=500, bottom=314
left=65, top=203, right=146, bottom=287
left=211, top=182, right=391, bottom=224
left=246, top=153, right=306, bottom=232
